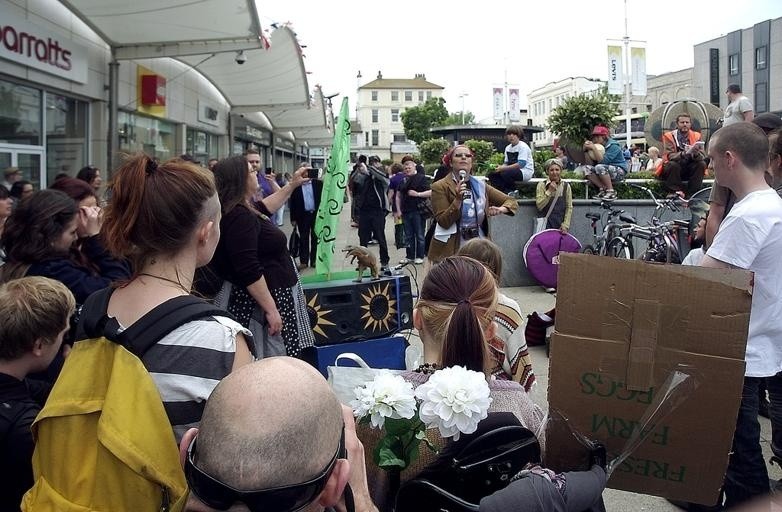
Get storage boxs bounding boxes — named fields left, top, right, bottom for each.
left=544, top=252, right=754, bottom=506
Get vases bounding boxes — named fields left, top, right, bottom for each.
left=373, top=466, right=399, bottom=512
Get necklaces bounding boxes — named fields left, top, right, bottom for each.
left=412, top=363, right=441, bottom=374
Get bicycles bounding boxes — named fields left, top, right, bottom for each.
left=579, top=197, right=625, bottom=255
left=608, top=184, right=690, bottom=267
left=379, top=258, right=420, bottom=343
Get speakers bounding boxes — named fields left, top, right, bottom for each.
left=302, top=274, right=414, bottom=346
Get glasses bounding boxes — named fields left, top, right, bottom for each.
left=88, top=164, right=95, bottom=171
left=183, top=433, right=344, bottom=512
left=456, top=153, right=473, bottom=158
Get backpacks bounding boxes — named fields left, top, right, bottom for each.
left=20, top=286, right=236, bottom=512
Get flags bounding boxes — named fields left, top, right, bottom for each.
left=313, top=97, right=351, bottom=271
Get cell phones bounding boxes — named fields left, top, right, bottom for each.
left=307, top=169, right=319, bottom=179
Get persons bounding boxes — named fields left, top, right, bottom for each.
left=241, top=150, right=281, bottom=225
left=10, top=180, right=33, bottom=204
left=276, top=172, right=287, bottom=226
left=76, top=166, right=102, bottom=189
left=701, top=121, right=782, bottom=512
left=427, top=144, right=520, bottom=265
left=352, top=155, right=391, bottom=270
left=212, top=155, right=317, bottom=360
left=488, top=125, right=534, bottom=199
left=655, top=113, right=707, bottom=197
left=349, top=154, right=368, bottom=227
left=456, top=236, right=537, bottom=394
left=178, top=355, right=379, bottom=512
left=1, top=166, right=24, bottom=191
left=0, top=274, right=77, bottom=512
left=705, top=111, right=782, bottom=418
left=48, top=177, right=97, bottom=208
left=646, top=146, right=662, bottom=172
left=395, top=161, right=431, bottom=264
left=0, top=188, right=137, bottom=382
left=629, top=144, right=636, bottom=157
left=555, top=145, right=568, bottom=168
left=0, top=183, right=14, bottom=268
left=387, top=163, right=405, bottom=248
left=290, top=161, right=323, bottom=269
left=18, top=154, right=258, bottom=512
left=630, top=151, right=642, bottom=173
left=355, top=255, right=546, bottom=512
left=535, top=158, right=573, bottom=293
left=583, top=123, right=629, bottom=201
left=722, top=83, right=754, bottom=127
left=681, top=210, right=709, bottom=266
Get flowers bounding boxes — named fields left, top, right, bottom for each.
left=352, top=366, right=492, bottom=467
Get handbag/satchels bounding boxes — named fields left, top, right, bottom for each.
left=288, top=226, right=300, bottom=258
left=533, top=182, right=563, bottom=235
left=415, top=197, right=433, bottom=220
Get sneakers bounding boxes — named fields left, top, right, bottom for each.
left=399, top=257, right=413, bottom=264
left=415, top=257, right=424, bottom=264
left=541, top=284, right=556, bottom=294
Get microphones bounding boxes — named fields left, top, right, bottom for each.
left=459, top=170, right=466, bottom=182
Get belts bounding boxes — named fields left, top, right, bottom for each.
left=305, top=210, right=316, bottom=214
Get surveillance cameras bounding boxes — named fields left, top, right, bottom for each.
left=233, top=52, right=248, bottom=66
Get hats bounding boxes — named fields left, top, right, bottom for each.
left=750, top=112, right=782, bottom=129
left=592, top=126, right=609, bottom=135
left=3, top=166, right=20, bottom=176
left=181, top=153, right=200, bottom=163
